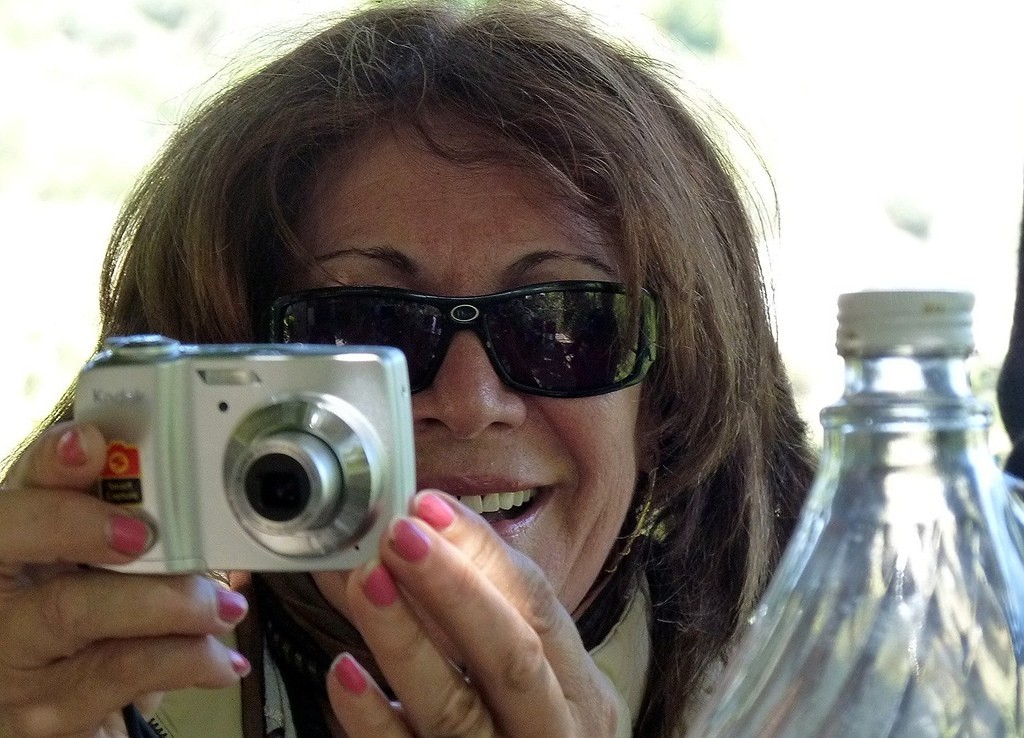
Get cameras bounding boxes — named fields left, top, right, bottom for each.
left=73, top=335, right=417, bottom=576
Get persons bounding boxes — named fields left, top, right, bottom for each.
left=3, top=0, right=831, bottom=738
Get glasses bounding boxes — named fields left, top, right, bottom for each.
left=253, top=280, right=665, bottom=399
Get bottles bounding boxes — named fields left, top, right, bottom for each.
left=694, top=290, right=1024, bottom=738
